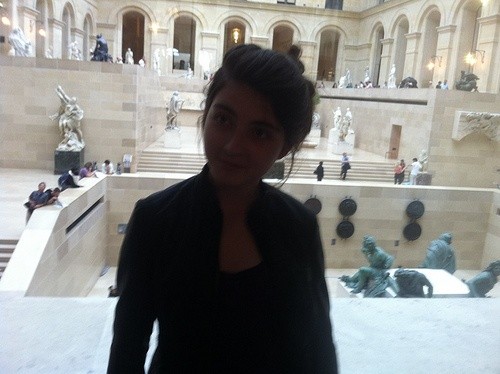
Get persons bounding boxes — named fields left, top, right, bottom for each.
left=343, top=67, right=352, bottom=83
left=313, top=161, right=323, bottom=181
left=125, top=48, right=134, bottom=64
left=312, top=106, right=353, bottom=141
left=362, top=65, right=370, bottom=85
left=91, top=33, right=108, bottom=62
left=436, top=80, right=448, bottom=90
left=58, top=97, right=86, bottom=145
left=166, top=91, right=185, bottom=129
left=105, top=44, right=339, bottom=374
left=338, top=232, right=500, bottom=298
left=202, top=72, right=214, bottom=80
left=24, top=152, right=131, bottom=224
left=314, top=80, right=387, bottom=89
left=387, top=63, right=397, bottom=87
left=9, top=24, right=82, bottom=61
left=394, top=157, right=422, bottom=185
left=339, top=152, right=351, bottom=180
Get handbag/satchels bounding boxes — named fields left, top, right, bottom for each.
left=343, top=164, right=350, bottom=169
left=315, top=166, right=320, bottom=174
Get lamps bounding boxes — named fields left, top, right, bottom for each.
left=426, top=55, right=442, bottom=70
left=465, top=50, right=485, bottom=64
